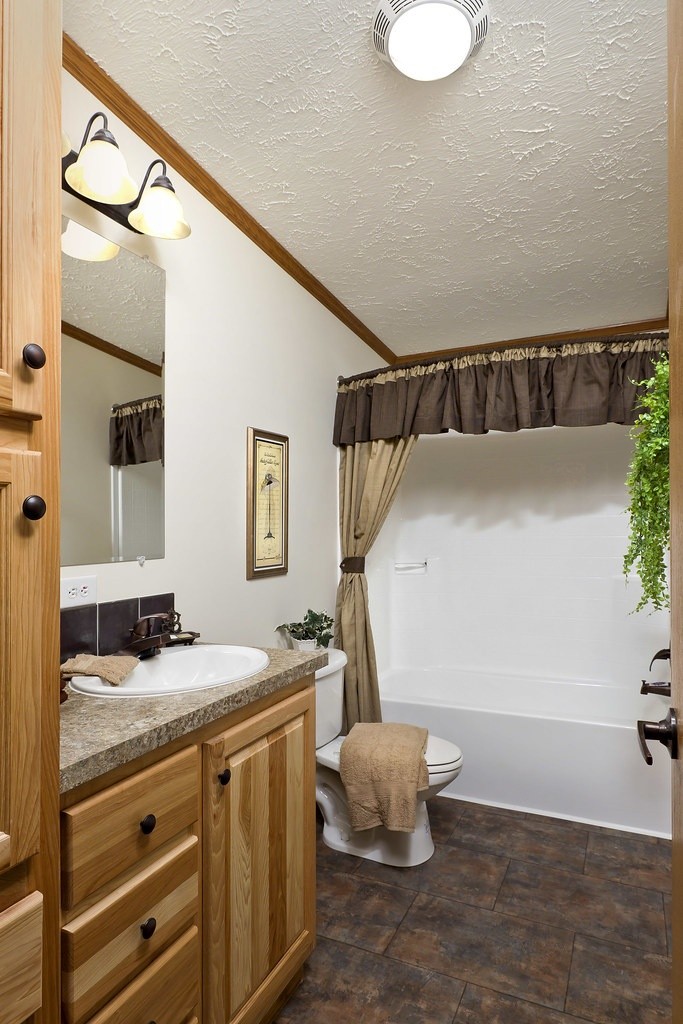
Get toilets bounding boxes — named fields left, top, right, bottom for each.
left=313, top=647, right=464, bottom=868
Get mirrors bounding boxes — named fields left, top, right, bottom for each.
left=58, top=222, right=171, bottom=570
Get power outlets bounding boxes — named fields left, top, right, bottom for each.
left=60, top=574, right=100, bottom=609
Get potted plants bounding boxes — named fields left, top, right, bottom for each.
left=274, top=608, right=335, bottom=653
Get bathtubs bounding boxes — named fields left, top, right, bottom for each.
left=374, top=665, right=672, bottom=840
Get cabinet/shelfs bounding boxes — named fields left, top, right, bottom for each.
left=63, top=674, right=325, bottom=1024
left=0, top=0, right=71, bottom=1024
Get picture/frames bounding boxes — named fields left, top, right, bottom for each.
left=244, top=427, right=295, bottom=580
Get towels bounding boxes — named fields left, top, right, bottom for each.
left=339, top=722, right=430, bottom=833
left=59, top=652, right=141, bottom=687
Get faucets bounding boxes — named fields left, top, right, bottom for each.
left=128, top=613, right=176, bottom=642
left=111, top=633, right=172, bottom=658
left=640, top=680, right=671, bottom=697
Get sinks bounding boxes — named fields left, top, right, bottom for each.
left=70, top=644, right=270, bottom=695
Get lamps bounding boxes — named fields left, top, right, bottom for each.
left=61, top=111, right=196, bottom=246
left=61, top=218, right=120, bottom=262
left=372, top=0, right=491, bottom=84
